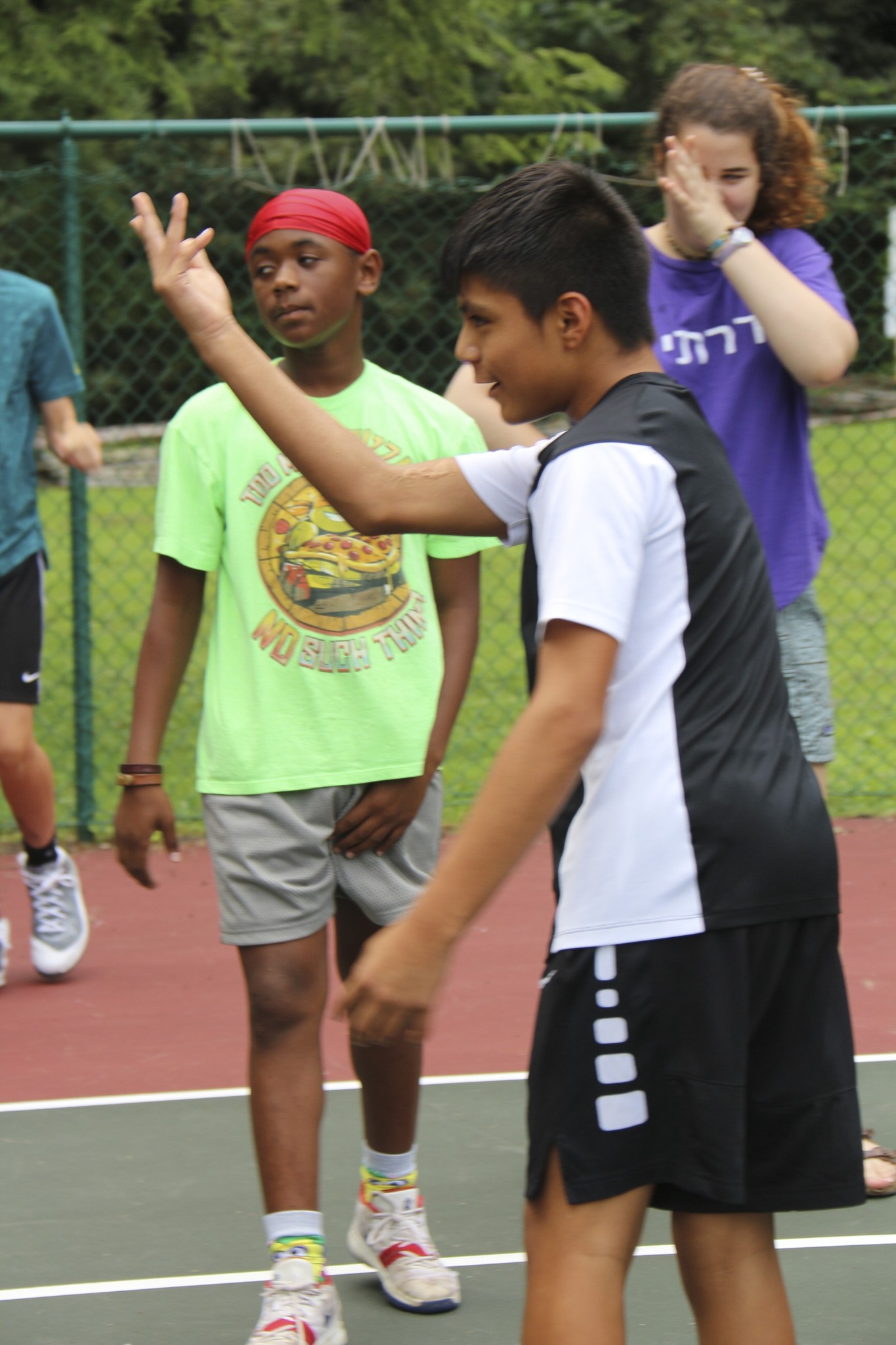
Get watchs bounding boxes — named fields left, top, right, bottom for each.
left=711, top=226, right=754, bottom=269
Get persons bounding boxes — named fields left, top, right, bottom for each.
left=115, top=186, right=488, bottom=1345
left=0, top=270, right=103, bottom=985
left=443, top=62, right=896, bottom=1198
left=130, top=156, right=865, bottom=1345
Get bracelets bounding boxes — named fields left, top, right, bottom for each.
left=114, top=764, right=162, bottom=787
left=706, top=221, right=744, bottom=252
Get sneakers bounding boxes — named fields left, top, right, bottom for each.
left=16, top=846, right=90, bottom=980
left=347, top=1186, right=461, bottom=1314
left=247, top=1259, right=348, bottom=1345
left=0, top=918, right=12, bottom=987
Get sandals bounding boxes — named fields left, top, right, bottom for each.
left=860, top=1129, right=896, bottom=1196
